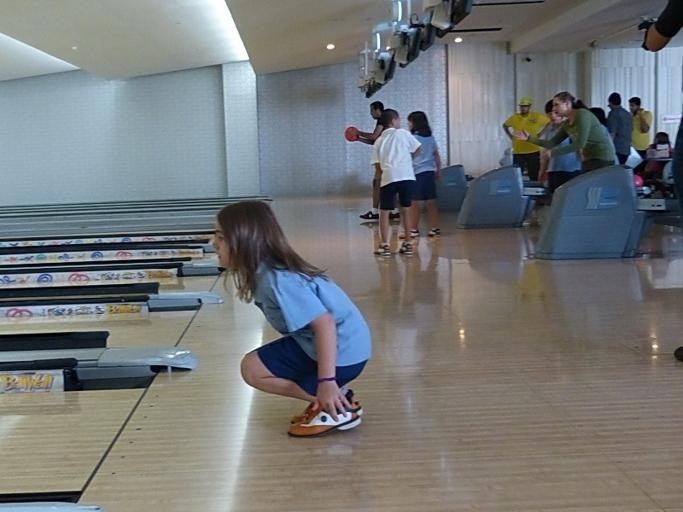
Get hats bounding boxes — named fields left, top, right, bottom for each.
left=519, top=96, right=533, bottom=106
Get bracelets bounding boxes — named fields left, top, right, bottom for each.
left=315, top=377, right=336, bottom=383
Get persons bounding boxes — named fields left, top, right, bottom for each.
left=399, top=111, right=441, bottom=241
left=502, top=92, right=675, bottom=210
left=359, top=101, right=400, bottom=221
left=370, top=108, right=423, bottom=259
left=645, top=0, right=682, bottom=366
left=214, top=200, right=371, bottom=436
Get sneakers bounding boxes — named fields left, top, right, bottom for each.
left=287, top=388, right=363, bottom=437
left=389, top=211, right=400, bottom=219
left=359, top=211, right=379, bottom=219
left=360, top=221, right=379, bottom=229
left=372, top=242, right=392, bottom=256
left=399, top=229, right=420, bottom=254
left=427, top=227, right=441, bottom=236
